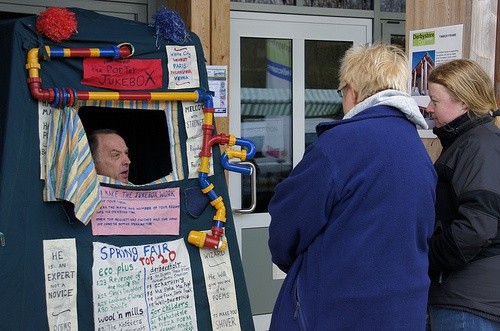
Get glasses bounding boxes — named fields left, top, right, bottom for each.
left=337, top=84, right=348, bottom=97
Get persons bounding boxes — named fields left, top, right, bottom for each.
left=266, top=39, right=440, bottom=331
left=423, top=59, right=500, bottom=331
left=86, top=125, right=131, bottom=185
